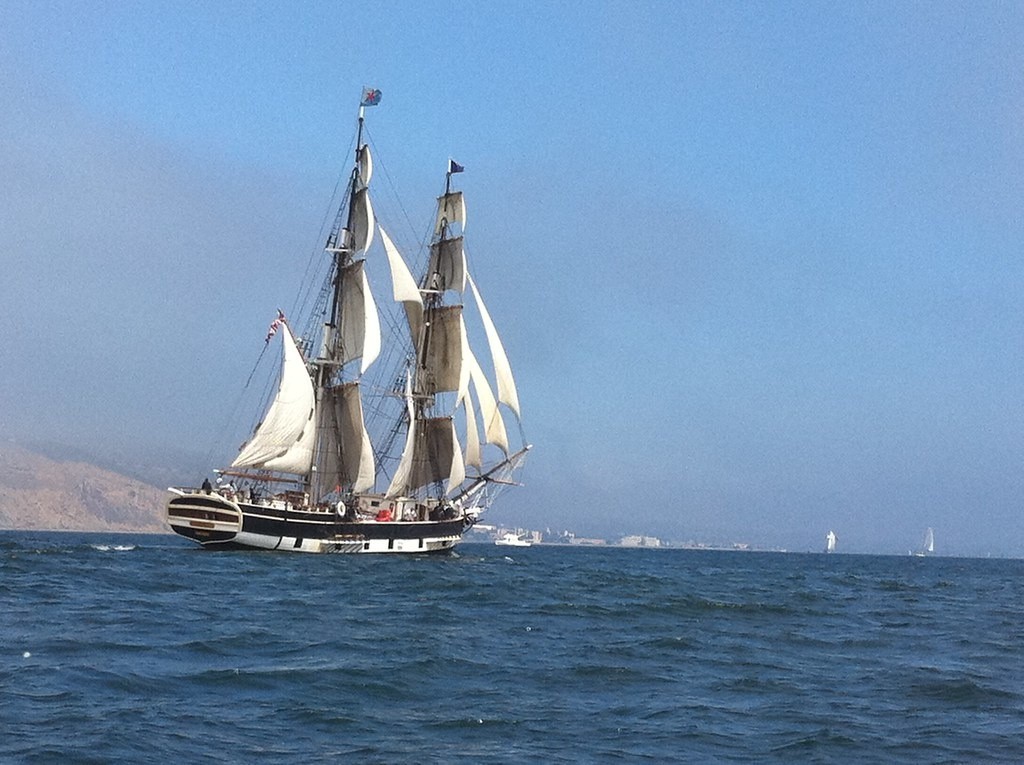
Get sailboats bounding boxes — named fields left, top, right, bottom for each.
left=166, top=83, right=535, bottom=556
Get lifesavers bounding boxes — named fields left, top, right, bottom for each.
left=375, top=509, right=390, bottom=521
left=338, top=501, right=346, bottom=516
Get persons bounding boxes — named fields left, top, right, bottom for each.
left=201, top=478, right=252, bottom=503
left=344, top=493, right=362, bottom=522
left=402, top=507, right=417, bottom=522
